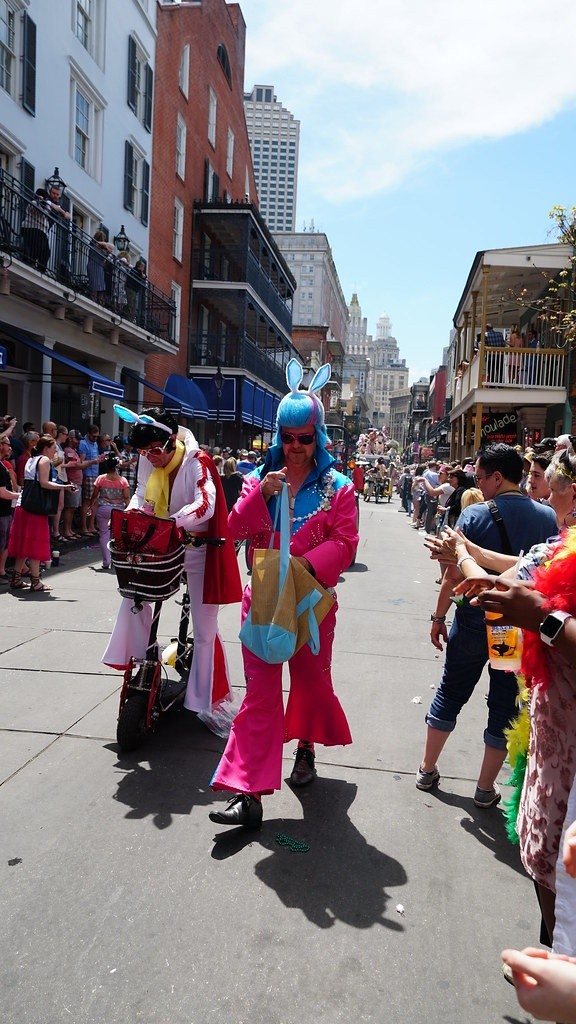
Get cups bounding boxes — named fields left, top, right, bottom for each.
left=45, top=560, right=52, bottom=569
left=58, top=450, right=65, bottom=461
left=80, top=453, right=86, bottom=461
left=67, top=453, right=74, bottom=461
left=52, top=551, right=60, bottom=567
left=482, top=600, right=522, bottom=670
left=11, top=498, right=17, bottom=507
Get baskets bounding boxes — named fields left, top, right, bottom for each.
left=107, top=541, right=187, bottom=600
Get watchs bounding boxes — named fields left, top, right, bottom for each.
left=539, top=611, right=572, bottom=647
left=457, top=556, right=476, bottom=575
left=431, top=613, right=446, bottom=622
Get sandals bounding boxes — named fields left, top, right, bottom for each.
left=30, top=575, right=52, bottom=591
left=10, top=570, right=30, bottom=588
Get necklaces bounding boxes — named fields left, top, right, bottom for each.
left=495, top=489, right=523, bottom=498
left=289, top=487, right=295, bottom=510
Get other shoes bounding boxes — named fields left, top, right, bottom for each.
left=408, top=520, right=423, bottom=529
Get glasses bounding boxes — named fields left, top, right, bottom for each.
left=473, top=473, right=491, bottom=484
left=105, top=438, right=112, bottom=442
left=281, top=432, right=315, bottom=445
left=91, top=434, right=99, bottom=438
left=136, top=439, right=170, bottom=456
left=438, top=472, right=445, bottom=474
left=3, top=442, right=11, bottom=447
left=62, top=432, right=68, bottom=436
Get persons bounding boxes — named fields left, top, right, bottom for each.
left=423, top=451, right=576, bottom=1024
left=365, top=457, right=397, bottom=503
left=87, top=459, right=130, bottom=569
left=105, top=407, right=241, bottom=739
left=398, top=434, right=576, bottom=586
left=457, top=323, right=543, bottom=388
left=9, top=435, right=80, bottom=593
left=0, top=414, right=138, bottom=560
left=415, top=442, right=560, bottom=810
left=21, top=185, right=72, bottom=273
left=0, top=435, right=22, bottom=584
left=201, top=442, right=265, bottom=515
left=86, top=230, right=148, bottom=323
left=208, top=391, right=360, bottom=826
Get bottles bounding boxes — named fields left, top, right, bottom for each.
left=136, top=500, right=156, bottom=514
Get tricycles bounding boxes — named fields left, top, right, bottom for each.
left=362, top=468, right=393, bottom=505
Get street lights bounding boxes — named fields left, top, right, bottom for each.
left=213, top=365, right=225, bottom=447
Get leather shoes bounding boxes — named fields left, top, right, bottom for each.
left=291, top=748, right=316, bottom=786
left=209, top=793, right=263, bottom=826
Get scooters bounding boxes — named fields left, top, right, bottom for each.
left=105, top=524, right=226, bottom=752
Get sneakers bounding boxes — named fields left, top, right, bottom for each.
left=475, top=781, right=501, bottom=807
left=415, top=761, right=439, bottom=789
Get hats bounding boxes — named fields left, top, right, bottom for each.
left=124, top=438, right=129, bottom=444
left=533, top=437, right=557, bottom=447
left=69, top=429, right=85, bottom=440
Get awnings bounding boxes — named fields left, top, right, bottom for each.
left=124, top=368, right=209, bottom=420
left=0, top=324, right=126, bottom=402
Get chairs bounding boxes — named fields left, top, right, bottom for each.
left=199, top=263, right=219, bottom=280
left=1, top=216, right=23, bottom=259
left=194, top=343, right=211, bottom=365
left=56, top=257, right=89, bottom=288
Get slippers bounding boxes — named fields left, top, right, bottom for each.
left=1, top=572, right=11, bottom=579
left=66, top=532, right=81, bottom=540
left=82, top=530, right=94, bottom=537
left=54, top=534, right=69, bottom=542
left=22, top=569, right=41, bottom=577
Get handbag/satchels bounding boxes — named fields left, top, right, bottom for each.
left=239, top=483, right=336, bottom=665
left=111, top=508, right=178, bottom=556
left=21, top=455, right=61, bottom=514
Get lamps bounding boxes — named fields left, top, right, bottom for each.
left=113, top=224, right=131, bottom=251
left=45, top=166, right=68, bottom=200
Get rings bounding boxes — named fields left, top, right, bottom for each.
left=273, top=491, right=279, bottom=496
